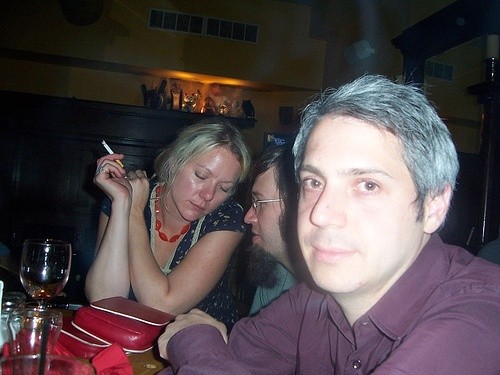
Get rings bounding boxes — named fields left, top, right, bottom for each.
left=99, top=166, right=103, bottom=171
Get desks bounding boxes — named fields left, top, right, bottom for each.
left=30, top=306, right=169, bottom=374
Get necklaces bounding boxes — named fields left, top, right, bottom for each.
left=153, top=186, right=190, bottom=243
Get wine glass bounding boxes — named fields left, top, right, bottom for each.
left=19, top=236, right=72, bottom=308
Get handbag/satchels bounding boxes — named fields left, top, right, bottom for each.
left=51, top=297, right=176, bottom=358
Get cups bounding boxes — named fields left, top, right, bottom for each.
left=0, top=292, right=63, bottom=375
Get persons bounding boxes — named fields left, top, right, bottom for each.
left=83, top=117, right=251, bottom=337
left=244, top=142, right=305, bottom=318
left=158, top=75, right=499, bottom=375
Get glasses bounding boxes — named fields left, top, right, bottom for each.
left=250, top=193, right=283, bottom=209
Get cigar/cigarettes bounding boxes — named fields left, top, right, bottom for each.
left=101, top=140, right=124, bottom=167
